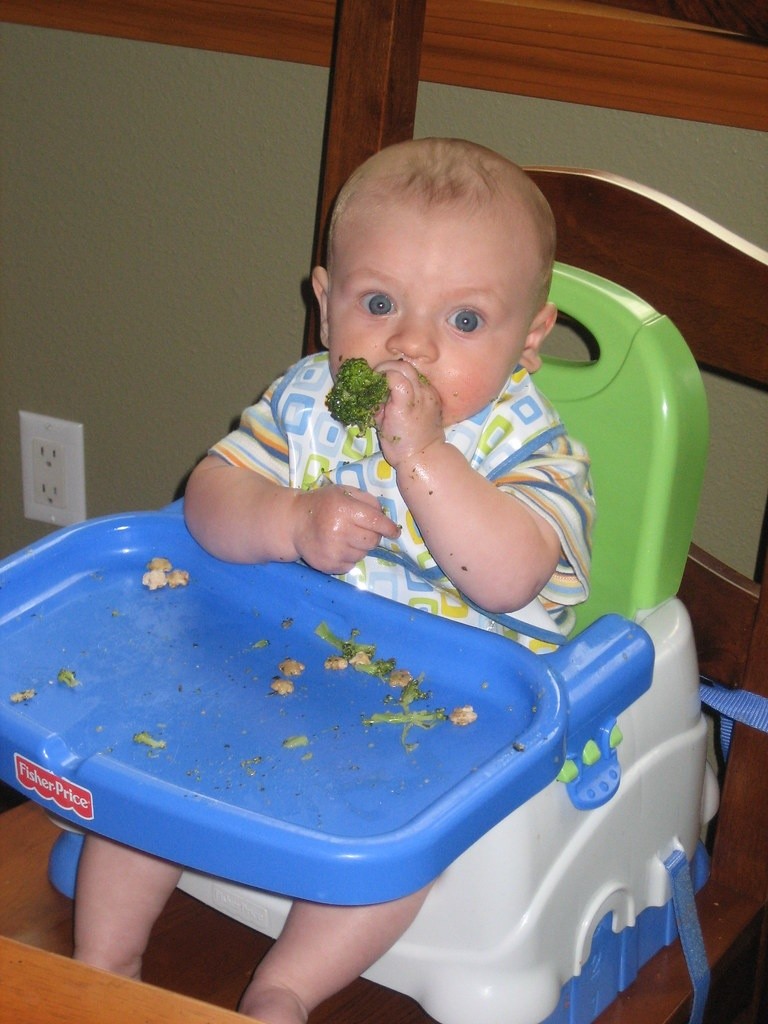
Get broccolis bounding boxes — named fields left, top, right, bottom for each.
left=325, top=358, right=388, bottom=427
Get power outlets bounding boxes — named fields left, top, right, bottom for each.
left=18, top=410, right=86, bottom=526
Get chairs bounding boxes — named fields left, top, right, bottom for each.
left=0, top=257, right=720, bottom=1024
left=142, top=0, right=767, bottom=1024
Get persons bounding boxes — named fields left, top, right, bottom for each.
left=69, top=138, right=597, bottom=1022
